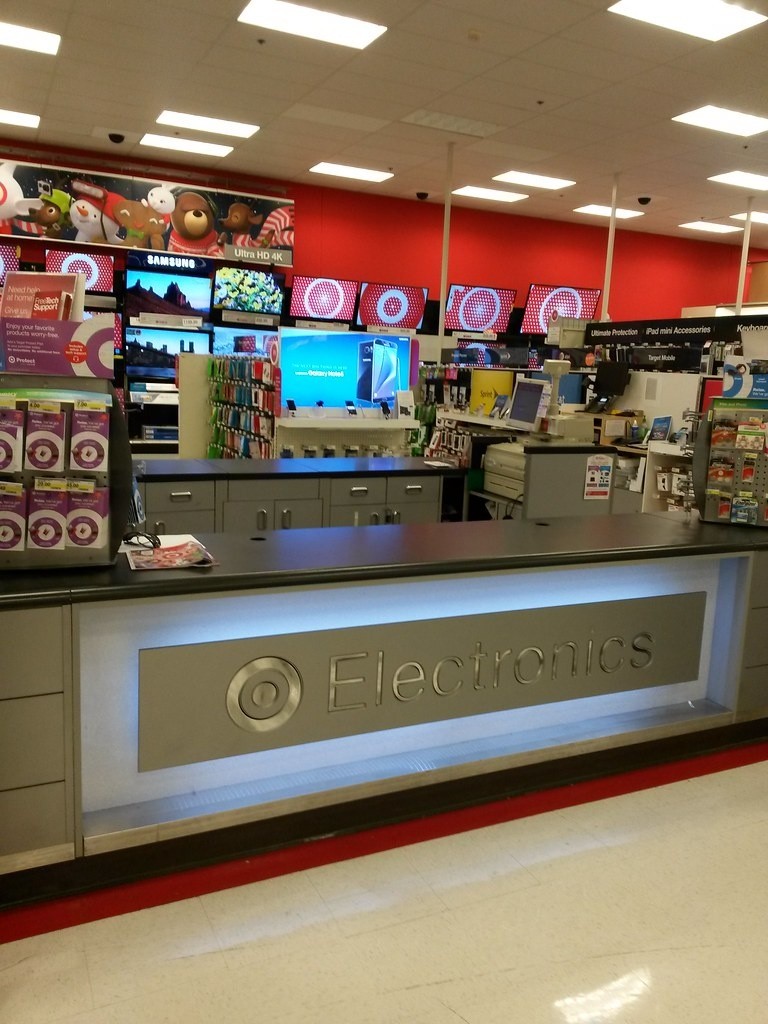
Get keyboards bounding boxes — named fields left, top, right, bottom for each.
left=487, top=441, right=534, bottom=458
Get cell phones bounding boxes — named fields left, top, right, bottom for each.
left=345, top=401, right=357, bottom=415
left=287, top=400, right=296, bottom=411
left=380, top=401, right=391, bottom=414
left=355, top=338, right=400, bottom=405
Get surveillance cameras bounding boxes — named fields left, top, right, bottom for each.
left=638, top=197, right=651, bottom=205
left=108, top=133, right=125, bottom=143
left=416, top=192, right=428, bottom=200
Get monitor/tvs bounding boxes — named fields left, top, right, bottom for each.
left=505, top=377, right=548, bottom=433
left=593, top=361, right=631, bottom=395
left=122, top=249, right=287, bottom=401
left=444, top=284, right=517, bottom=337
left=356, top=282, right=429, bottom=330
left=0, top=243, right=20, bottom=287
left=289, top=274, right=359, bottom=324
left=45, top=249, right=114, bottom=295
left=520, top=284, right=602, bottom=334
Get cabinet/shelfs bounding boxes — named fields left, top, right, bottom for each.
left=136, top=456, right=459, bottom=534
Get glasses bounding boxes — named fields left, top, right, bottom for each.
left=123, top=531, right=161, bottom=549
